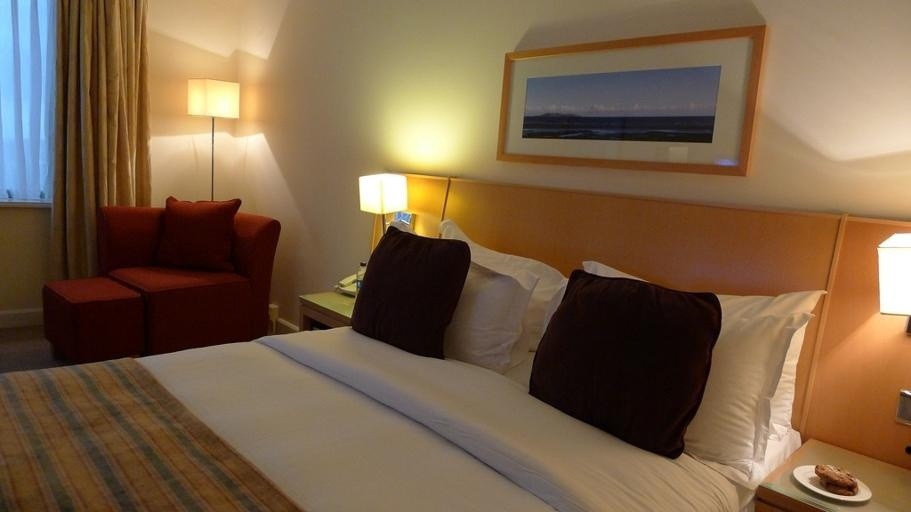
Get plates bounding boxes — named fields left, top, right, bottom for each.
left=792, top=464, right=873, bottom=505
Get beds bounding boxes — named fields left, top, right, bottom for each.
left=3, top=171, right=911, bottom=509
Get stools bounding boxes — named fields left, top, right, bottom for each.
left=44, top=275, right=147, bottom=365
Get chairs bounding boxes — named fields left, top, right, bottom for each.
left=97, top=204, right=281, bottom=357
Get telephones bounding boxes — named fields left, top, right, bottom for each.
left=337, top=271, right=364, bottom=297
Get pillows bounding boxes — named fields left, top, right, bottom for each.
left=583, top=259, right=828, bottom=444
left=160, top=193, right=245, bottom=275
left=351, top=224, right=470, bottom=361
left=444, top=258, right=539, bottom=375
left=438, top=218, right=566, bottom=354
left=684, top=313, right=815, bottom=485
left=527, top=268, right=723, bottom=460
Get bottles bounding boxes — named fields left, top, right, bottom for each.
left=355, top=261, right=366, bottom=296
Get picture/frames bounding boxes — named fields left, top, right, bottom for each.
left=495, top=25, right=768, bottom=178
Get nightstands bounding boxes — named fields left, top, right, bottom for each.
left=752, top=437, right=911, bottom=509
left=299, top=290, right=357, bottom=331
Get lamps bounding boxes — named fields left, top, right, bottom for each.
left=186, top=78, right=240, bottom=202
left=878, top=227, right=911, bottom=456
left=356, top=173, right=408, bottom=237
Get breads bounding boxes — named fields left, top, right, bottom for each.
left=815, top=464, right=858, bottom=487
left=819, top=480, right=858, bottom=496
